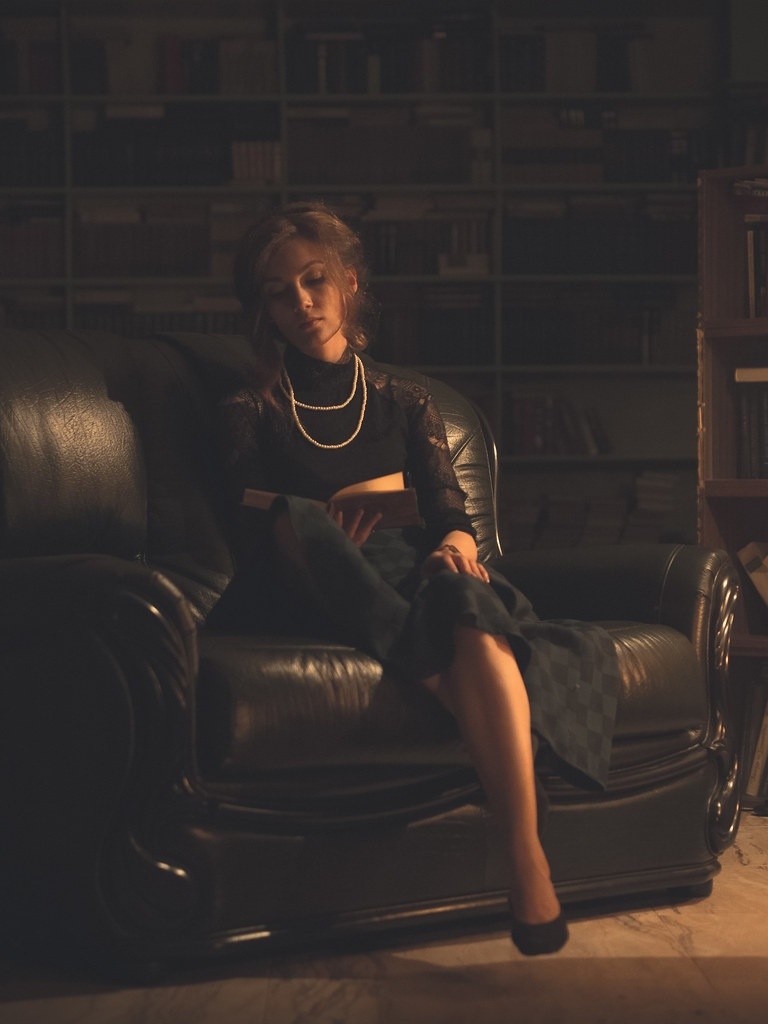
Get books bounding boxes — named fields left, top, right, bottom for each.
left=239, top=471, right=421, bottom=529
left=0, top=33, right=768, bottom=554
left=736, top=679, right=768, bottom=810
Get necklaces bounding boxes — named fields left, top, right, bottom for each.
left=282, top=357, right=367, bottom=450
left=277, top=351, right=360, bottom=411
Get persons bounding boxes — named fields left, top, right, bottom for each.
left=209, top=208, right=620, bottom=956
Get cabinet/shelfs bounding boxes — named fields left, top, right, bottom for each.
left=0, top=0, right=732, bottom=539
left=694, top=167, right=768, bottom=810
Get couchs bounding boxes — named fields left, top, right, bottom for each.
left=0, top=326, right=757, bottom=984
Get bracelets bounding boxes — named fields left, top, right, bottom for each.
left=439, top=544, right=462, bottom=556
